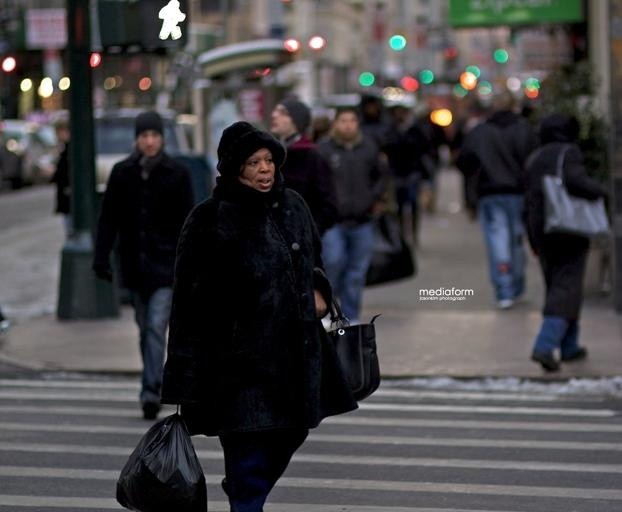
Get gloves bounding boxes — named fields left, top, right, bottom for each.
left=95, top=261, right=113, bottom=283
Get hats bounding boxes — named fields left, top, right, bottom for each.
left=134, top=111, right=164, bottom=138
left=281, top=97, right=310, bottom=131
left=217, top=122, right=286, bottom=184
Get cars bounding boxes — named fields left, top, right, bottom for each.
left=0, top=103, right=196, bottom=206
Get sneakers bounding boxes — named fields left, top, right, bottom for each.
left=498, top=298, right=513, bottom=310
left=564, top=346, right=586, bottom=360
left=533, top=349, right=559, bottom=369
left=141, top=388, right=160, bottom=419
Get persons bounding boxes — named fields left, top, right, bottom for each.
left=91, top=108, right=196, bottom=418
left=269, top=87, right=612, bottom=371
left=159, top=119, right=335, bottom=512
left=47, top=121, right=72, bottom=238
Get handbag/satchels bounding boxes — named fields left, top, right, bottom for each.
left=419, top=160, right=435, bottom=181
left=367, top=237, right=413, bottom=286
left=325, top=310, right=381, bottom=401
left=542, top=175, right=610, bottom=237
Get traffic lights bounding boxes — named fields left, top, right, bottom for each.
left=97, top=0, right=192, bottom=48
